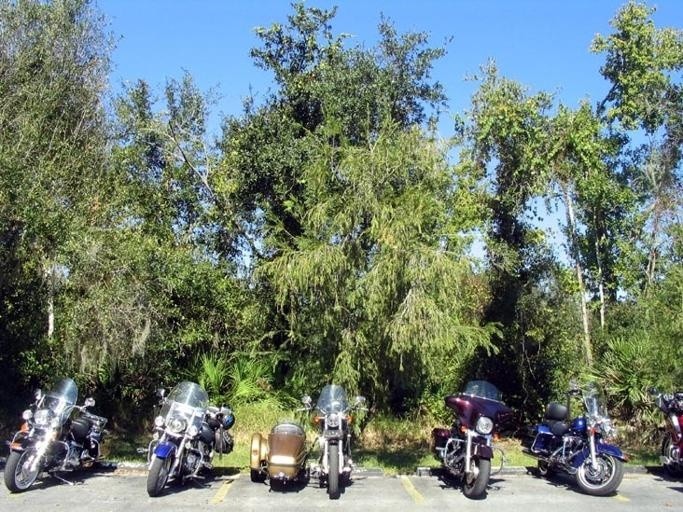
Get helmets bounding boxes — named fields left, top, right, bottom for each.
left=70, top=416, right=93, bottom=439
left=220, top=410, right=235, bottom=430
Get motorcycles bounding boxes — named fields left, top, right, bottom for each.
left=3, top=377, right=113, bottom=495
left=143, top=379, right=238, bottom=496
left=655, top=385, right=683, bottom=484
left=251, top=384, right=367, bottom=497
left=430, top=380, right=514, bottom=500
left=523, top=382, right=630, bottom=495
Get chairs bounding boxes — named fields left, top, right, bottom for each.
left=546, top=402, right=569, bottom=433
